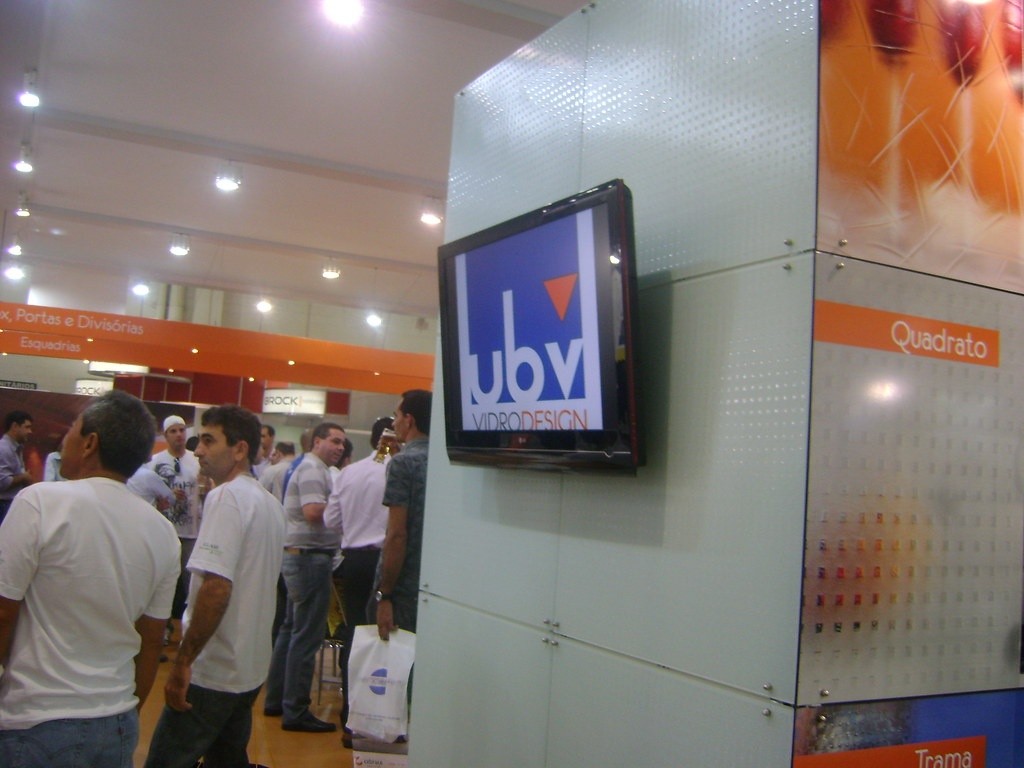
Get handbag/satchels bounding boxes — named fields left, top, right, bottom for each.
left=346, top=625, right=416, bottom=744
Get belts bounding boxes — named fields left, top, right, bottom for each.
left=286, top=546, right=335, bottom=555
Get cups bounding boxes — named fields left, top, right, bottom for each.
left=373, top=428, right=396, bottom=464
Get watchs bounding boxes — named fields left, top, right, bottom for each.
left=375, top=591, right=392, bottom=603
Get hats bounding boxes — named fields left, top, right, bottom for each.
left=162, top=414, right=185, bottom=434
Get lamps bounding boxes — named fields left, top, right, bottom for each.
left=7, top=228, right=21, bottom=255
left=216, top=162, right=246, bottom=193
left=323, top=258, right=340, bottom=279
left=15, top=189, right=31, bottom=217
left=15, top=136, right=35, bottom=173
left=422, top=197, right=445, bottom=226
left=170, top=233, right=189, bottom=257
left=19, top=68, right=39, bottom=107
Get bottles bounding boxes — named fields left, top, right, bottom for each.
left=164, top=628, right=171, bottom=645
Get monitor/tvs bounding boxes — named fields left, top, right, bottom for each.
left=437, top=178, right=647, bottom=477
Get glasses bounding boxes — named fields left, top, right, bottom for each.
left=174, top=457, right=180, bottom=474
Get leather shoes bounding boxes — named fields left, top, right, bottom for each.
left=265, top=708, right=283, bottom=716
left=281, top=711, right=336, bottom=733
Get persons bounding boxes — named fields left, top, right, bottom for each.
left=0, top=389, right=182, bottom=768
left=264, top=423, right=345, bottom=733
left=43, top=442, right=66, bottom=482
left=327, top=439, right=354, bottom=638
left=0, top=411, right=34, bottom=523
left=127, top=414, right=215, bottom=663
left=323, top=417, right=394, bottom=732
left=252, top=424, right=314, bottom=649
left=143, top=405, right=288, bottom=768
left=375, top=389, right=433, bottom=641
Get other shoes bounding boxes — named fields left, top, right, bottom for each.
left=164, top=623, right=172, bottom=645
left=160, top=654, right=167, bottom=663
